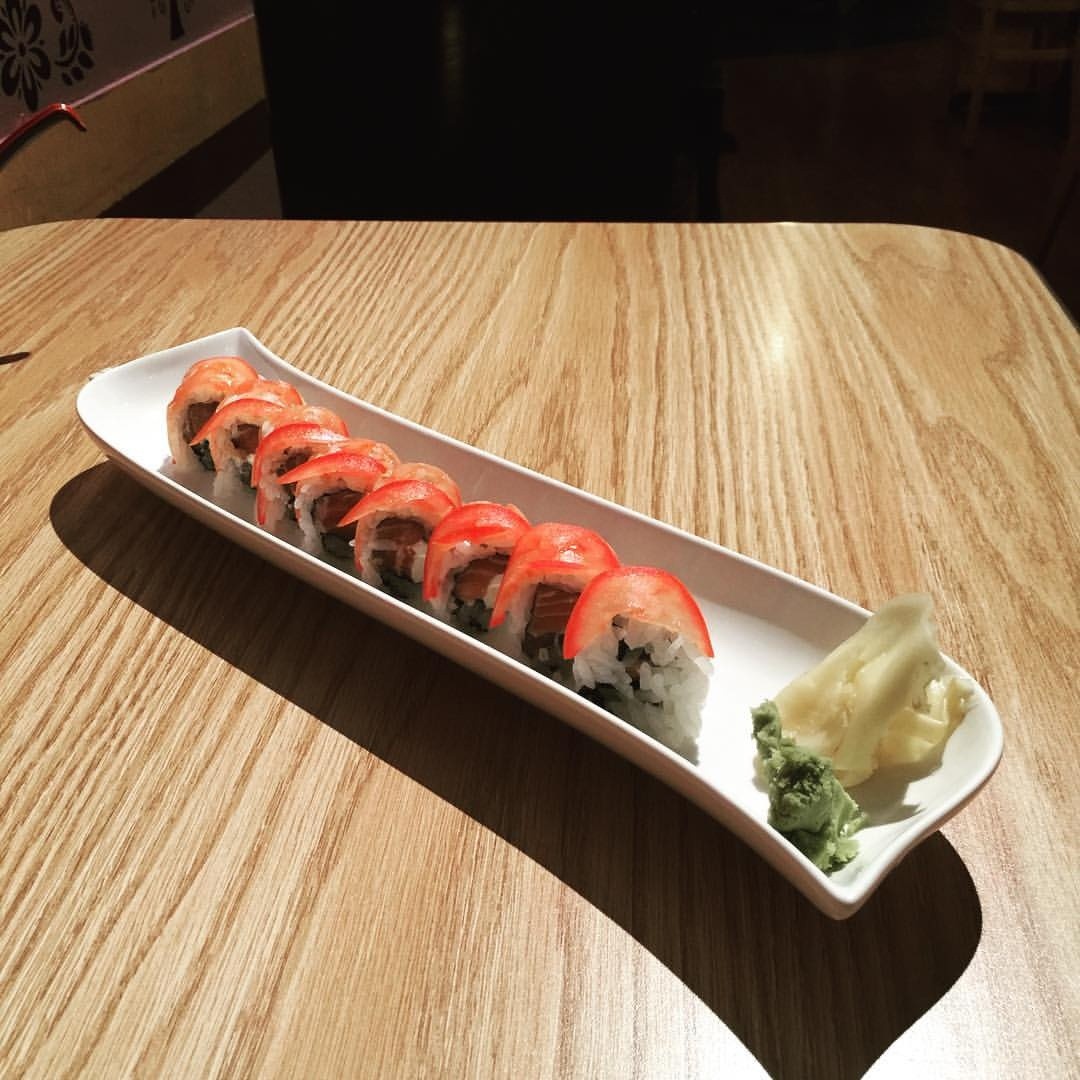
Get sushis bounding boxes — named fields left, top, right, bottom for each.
left=165, top=355, right=716, bottom=756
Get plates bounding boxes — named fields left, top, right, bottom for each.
left=73, top=326, right=1007, bottom=925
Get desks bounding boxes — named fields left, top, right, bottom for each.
left=0, top=216, right=1080, bottom=1080
